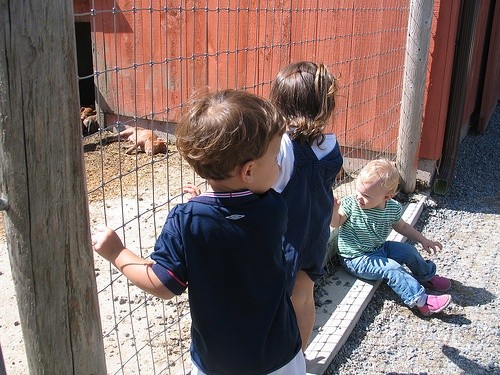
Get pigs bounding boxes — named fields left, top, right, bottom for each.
left=107, top=126, right=169, bottom=156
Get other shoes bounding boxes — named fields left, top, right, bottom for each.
left=422, top=275, right=451, bottom=291
left=417, top=294, right=451, bottom=317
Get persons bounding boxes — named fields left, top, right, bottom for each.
left=182, top=61, right=343, bottom=362
left=330, top=159, right=452, bottom=316
left=92, top=89, right=307, bottom=375
